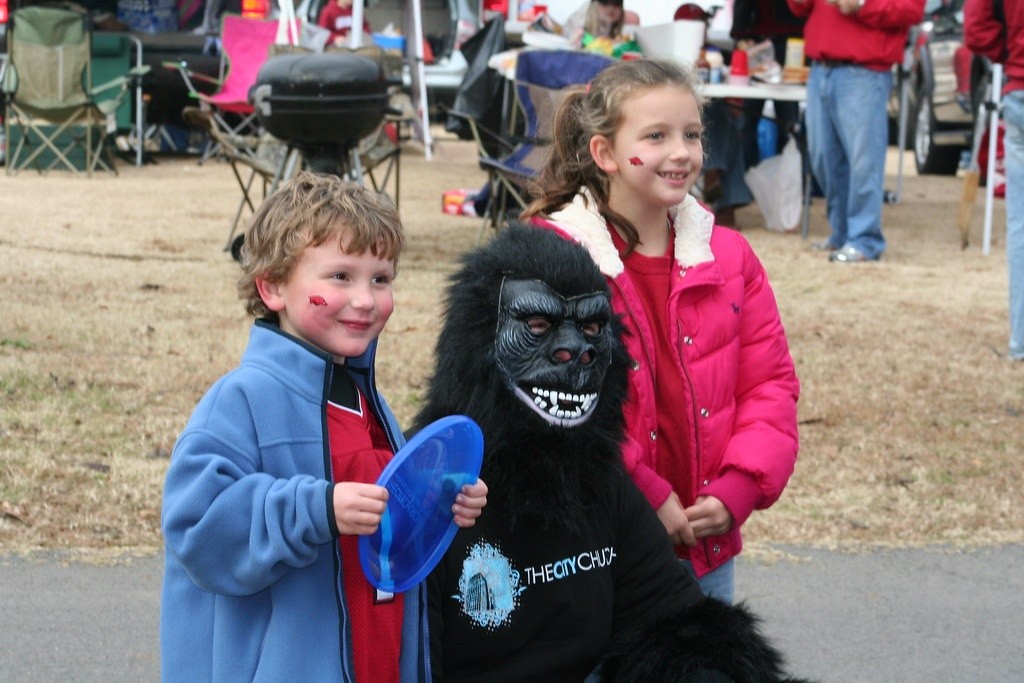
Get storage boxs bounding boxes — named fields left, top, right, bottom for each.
left=635, top=20, right=706, bottom=70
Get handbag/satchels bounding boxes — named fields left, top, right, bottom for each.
left=744, top=134, right=804, bottom=232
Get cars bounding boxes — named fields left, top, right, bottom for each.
left=291, top=1, right=481, bottom=101
left=887, top=0, right=984, bottom=178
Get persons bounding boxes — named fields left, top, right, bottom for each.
left=103, top=0, right=1024, bottom=360
left=527, top=58, right=798, bottom=607
left=160, top=171, right=488, bottom=683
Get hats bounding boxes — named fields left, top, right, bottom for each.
left=954, top=47, right=973, bottom=92
left=675, top=4, right=711, bottom=19
left=242, top=0, right=270, bottom=18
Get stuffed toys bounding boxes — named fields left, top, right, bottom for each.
left=405, top=225, right=811, bottom=683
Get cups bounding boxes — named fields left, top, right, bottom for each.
left=784, top=39, right=806, bottom=67
left=729, top=50, right=749, bottom=85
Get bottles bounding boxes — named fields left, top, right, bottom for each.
left=694, top=50, right=710, bottom=83
left=705, top=46, right=724, bottom=84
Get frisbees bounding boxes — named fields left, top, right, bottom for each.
left=358, top=415, right=484, bottom=593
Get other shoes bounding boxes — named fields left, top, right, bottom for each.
left=829, top=246, right=867, bottom=263
left=809, top=236, right=834, bottom=255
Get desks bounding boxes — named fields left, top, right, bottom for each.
left=95, top=30, right=218, bottom=54
left=402, top=65, right=469, bottom=88
left=697, top=83, right=812, bottom=241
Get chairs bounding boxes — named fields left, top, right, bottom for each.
left=448, top=48, right=621, bottom=250
left=0, top=6, right=416, bottom=253
